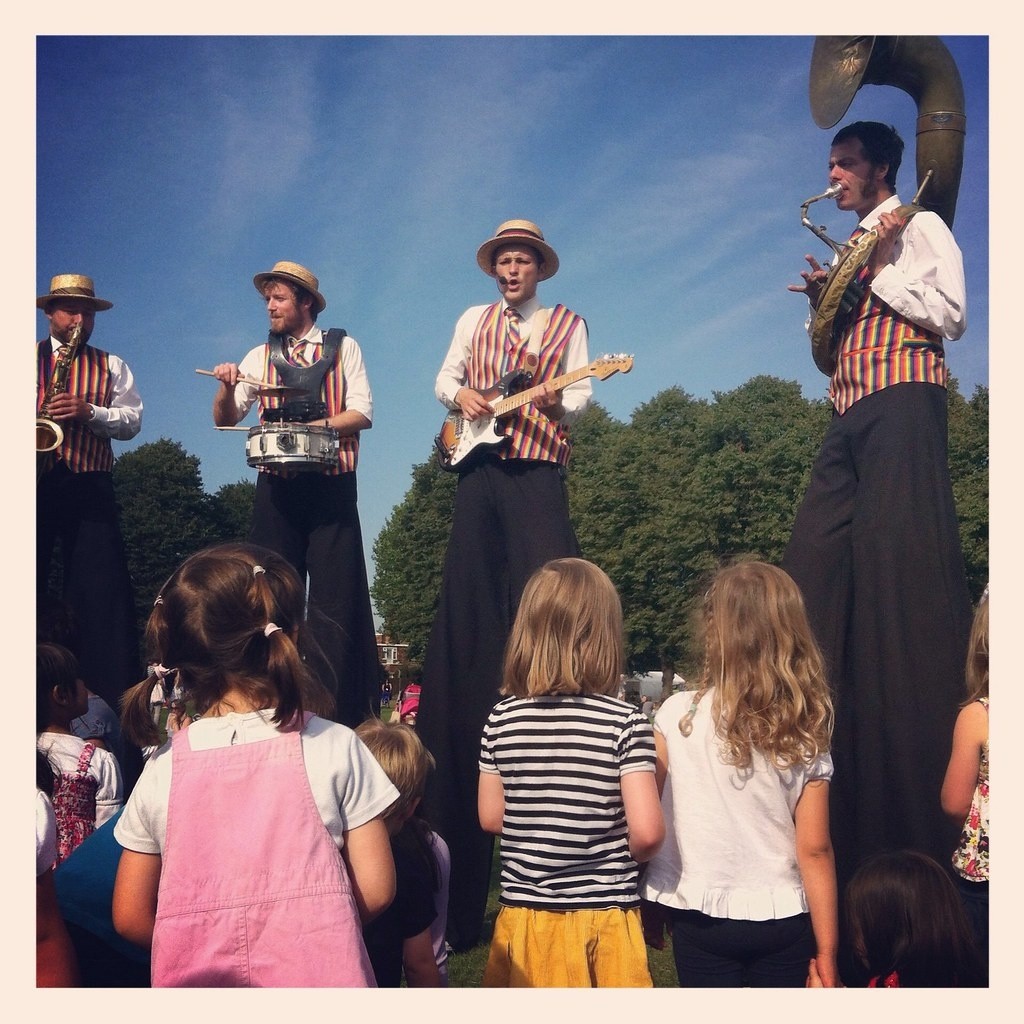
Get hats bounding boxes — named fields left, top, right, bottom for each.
left=254, top=261, right=327, bottom=314
left=478, top=220, right=560, bottom=284
left=37, top=274, right=114, bottom=312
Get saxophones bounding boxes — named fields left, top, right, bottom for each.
left=36, top=319, right=85, bottom=474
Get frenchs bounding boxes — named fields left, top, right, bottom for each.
left=804, top=35, right=967, bottom=375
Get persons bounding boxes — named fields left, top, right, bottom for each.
left=411, top=824, right=451, bottom=985
left=37, top=744, right=64, bottom=802
left=765, top=121, right=976, bottom=760
left=111, top=538, right=402, bottom=986
left=37, top=641, right=124, bottom=868
left=211, top=260, right=382, bottom=732
left=37, top=788, right=82, bottom=989
left=412, top=219, right=598, bottom=791
left=380, top=677, right=423, bottom=728
left=615, top=672, right=664, bottom=718
left=148, top=666, right=195, bottom=740
left=476, top=558, right=665, bottom=989
left=938, top=584, right=989, bottom=959
left=846, top=844, right=988, bottom=988
left=352, top=700, right=445, bottom=989
left=36, top=273, right=146, bottom=719
left=633, top=562, right=842, bottom=989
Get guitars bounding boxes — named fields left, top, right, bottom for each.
left=433, top=349, right=634, bottom=471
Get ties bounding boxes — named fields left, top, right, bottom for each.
left=504, top=309, right=521, bottom=350
left=843, top=228, right=865, bottom=255
left=289, top=339, right=310, bottom=368
left=50, top=346, right=70, bottom=387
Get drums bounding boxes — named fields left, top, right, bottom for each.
left=244, top=425, right=344, bottom=470
